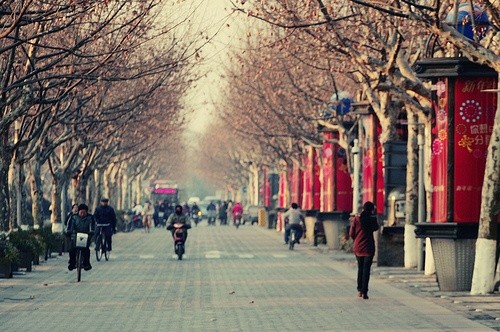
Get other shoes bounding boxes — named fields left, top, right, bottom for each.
left=286, top=240, right=288, bottom=244
left=68, top=265, right=76, bottom=271
left=108, top=245, right=111, bottom=251
left=357, top=291, right=361, bottom=297
left=362, top=294, right=368, bottom=299
left=95, top=248, right=100, bottom=251
left=297, top=240, right=300, bottom=244
left=85, top=266, right=92, bottom=270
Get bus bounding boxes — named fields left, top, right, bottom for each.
left=150, top=179, right=180, bottom=208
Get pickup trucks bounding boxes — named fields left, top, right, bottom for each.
left=242, top=204, right=265, bottom=225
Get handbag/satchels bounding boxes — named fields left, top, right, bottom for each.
left=76, top=233, right=88, bottom=248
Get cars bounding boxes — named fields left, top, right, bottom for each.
left=189, top=196, right=222, bottom=218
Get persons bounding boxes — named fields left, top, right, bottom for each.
left=94, top=199, right=117, bottom=251
left=165, top=205, right=191, bottom=244
left=284, top=203, right=306, bottom=244
left=64, top=203, right=95, bottom=271
left=121, top=199, right=242, bottom=232
left=351, top=201, right=378, bottom=299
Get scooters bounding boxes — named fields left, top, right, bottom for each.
left=191, top=209, right=199, bottom=226
left=172, top=222, right=188, bottom=261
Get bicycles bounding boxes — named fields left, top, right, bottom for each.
left=94, top=222, right=113, bottom=262
left=287, top=229, right=298, bottom=250
left=235, top=212, right=241, bottom=229
left=69, top=232, right=93, bottom=282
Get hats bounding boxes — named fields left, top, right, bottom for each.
left=363, top=202, right=374, bottom=210
left=101, top=199, right=108, bottom=202
left=78, top=204, right=88, bottom=211
